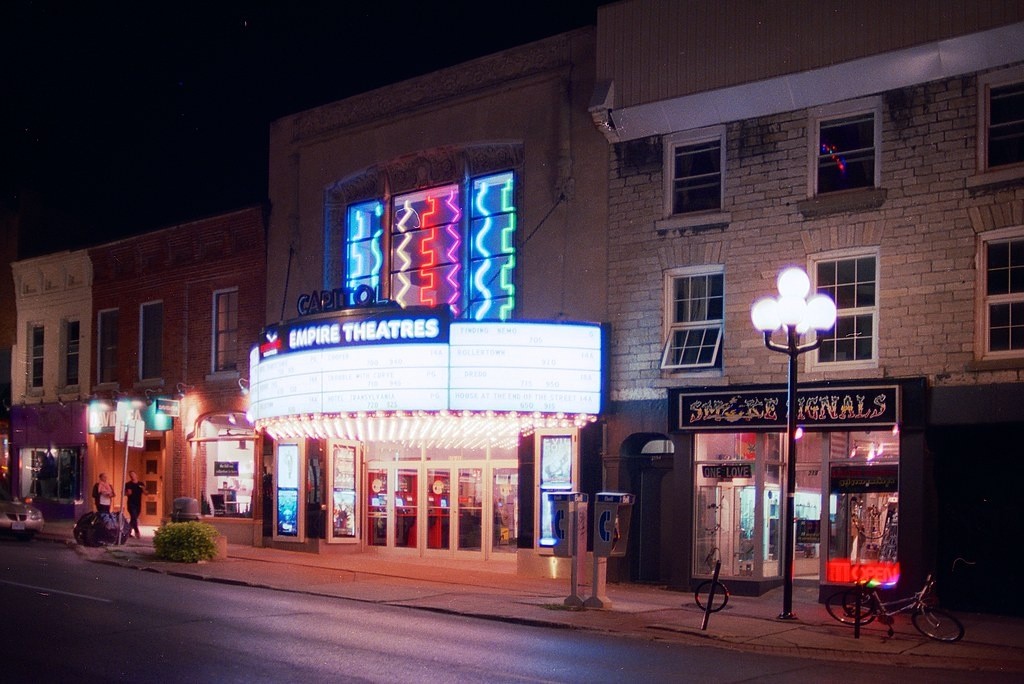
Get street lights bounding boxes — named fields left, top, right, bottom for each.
left=749, top=266, right=837, bottom=621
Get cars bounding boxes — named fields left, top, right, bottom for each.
left=0, top=486, right=45, bottom=543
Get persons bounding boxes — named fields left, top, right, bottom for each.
left=125, top=471, right=149, bottom=539
left=92, top=473, right=116, bottom=513
left=493, top=499, right=504, bottom=547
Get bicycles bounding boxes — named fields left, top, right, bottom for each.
left=825, top=573, right=967, bottom=644
